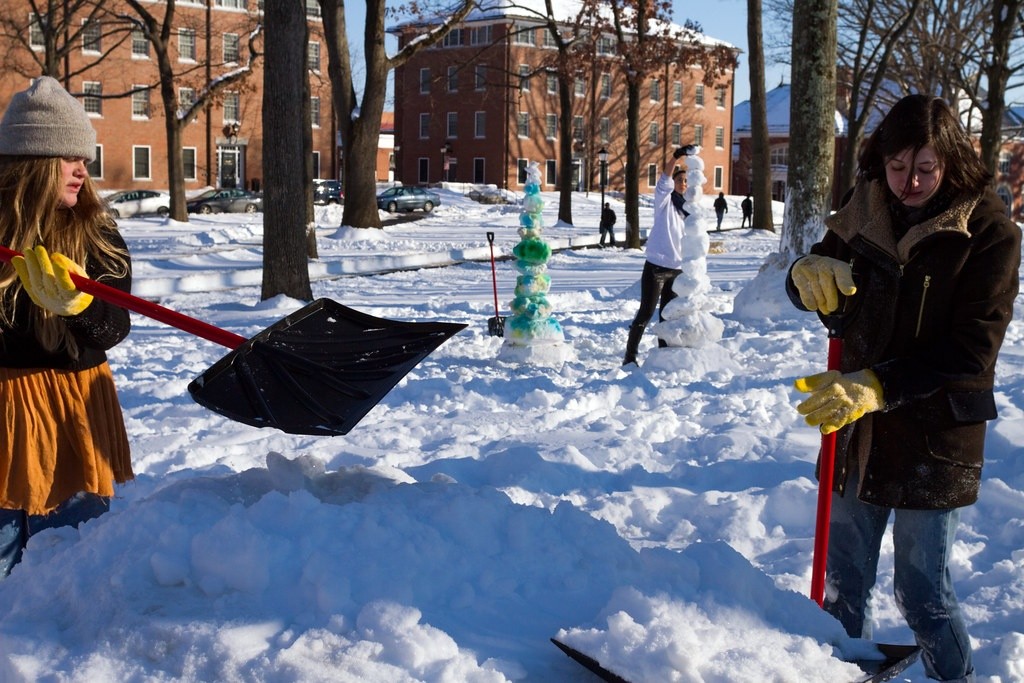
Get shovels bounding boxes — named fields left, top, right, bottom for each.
left=0, top=239, right=473, bottom=440
left=549, top=230, right=926, bottom=683
left=486, top=231, right=509, bottom=338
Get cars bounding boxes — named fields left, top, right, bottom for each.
left=312, top=180, right=361, bottom=202
left=376, top=186, right=444, bottom=212
left=185, top=188, right=266, bottom=214
left=104, top=189, right=178, bottom=218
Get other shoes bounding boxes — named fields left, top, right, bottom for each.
left=622, top=354, right=639, bottom=369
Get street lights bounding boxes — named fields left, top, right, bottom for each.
left=597, top=147, right=610, bottom=217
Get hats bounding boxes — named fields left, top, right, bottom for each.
left=1, top=74, right=97, bottom=164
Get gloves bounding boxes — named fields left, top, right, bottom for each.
left=792, top=252, right=858, bottom=317
left=11, top=244, right=97, bottom=316
left=794, top=366, right=886, bottom=435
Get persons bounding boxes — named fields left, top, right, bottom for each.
left=741, top=195, right=753, bottom=228
left=714, top=192, right=728, bottom=231
left=784, top=95, right=1022, bottom=683
left=598, top=203, right=617, bottom=248
left=0, top=74, right=135, bottom=518
left=622, top=144, right=698, bottom=367
